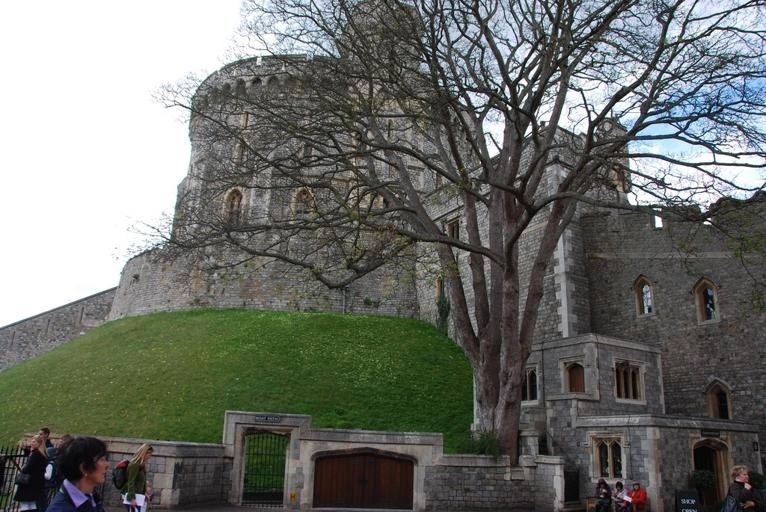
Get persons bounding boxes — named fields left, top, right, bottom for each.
left=729, top=464, right=762, bottom=512
left=594, top=478, right=648, bottom=512
left=12, top=427, right=110, bottom=512
left=120, top=443, right=155, bottom=512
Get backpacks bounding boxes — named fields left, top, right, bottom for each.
left=111, top=459, right=130, bottom=490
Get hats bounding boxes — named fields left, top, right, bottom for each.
left=45, top=447, right=55, bottom=457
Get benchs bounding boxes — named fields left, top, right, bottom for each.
left=586, top=496, right=650, bottom=512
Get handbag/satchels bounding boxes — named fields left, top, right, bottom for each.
left=720, top=495, right=744, bottom=511
left=122, top=492, right=146, bottom=507
left=14, top=472, right=32, bottom=486
